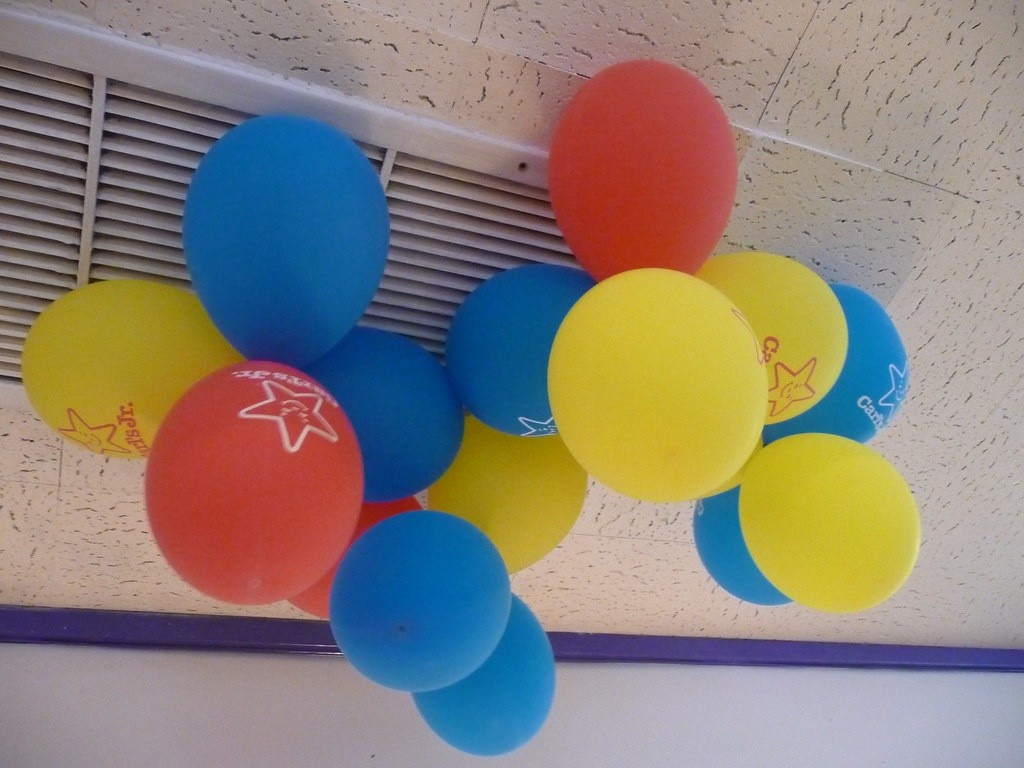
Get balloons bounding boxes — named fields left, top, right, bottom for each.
left=23, top=59, right=921, bottom=757
